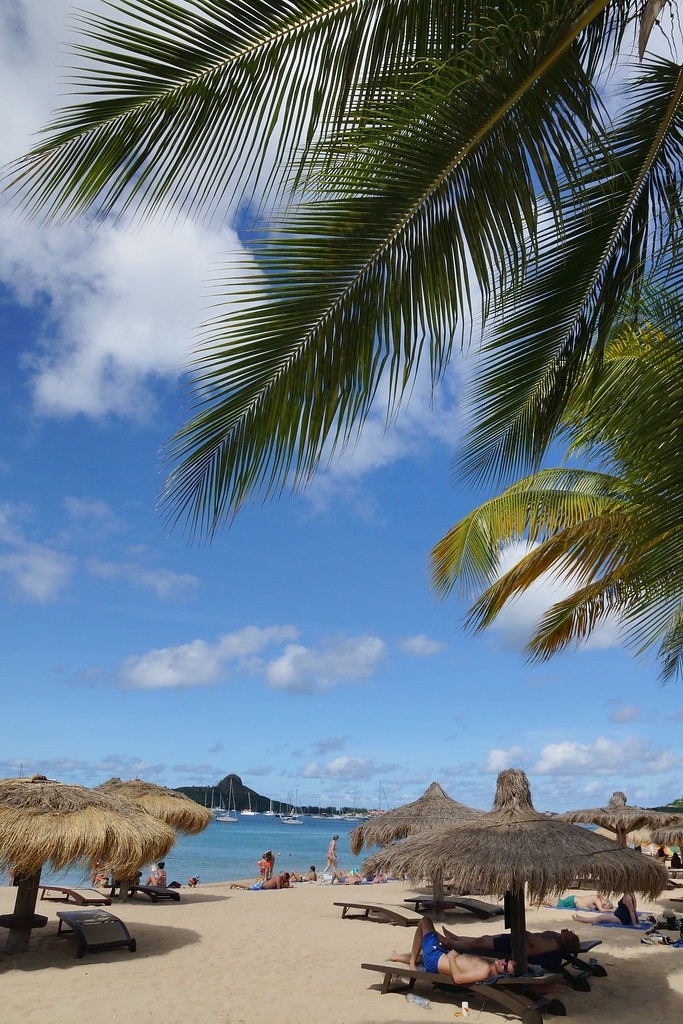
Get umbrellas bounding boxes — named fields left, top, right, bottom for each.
left=553, top=789, right=683, bottom=849
left=0, top=773, right=176, bottom=957
left=88, top=777, right=211, bottom=837
left=361, top=766, right=669, bottom=977
left=350, top=781, right=490, bottom=855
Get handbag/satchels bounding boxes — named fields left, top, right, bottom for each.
left=168, top=881, right=181, bottom=888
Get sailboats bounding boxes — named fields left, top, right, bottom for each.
left=265, top=791, right=304, bottom=824
left=216, top=778, right=238, bottom=822
left=240, top=793, right=259, bottom=815
left=202, top=789, right=226, bottom=812
left=313, top=781, right=388, bottom=823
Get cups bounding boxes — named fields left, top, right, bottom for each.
left=461, top=1001, right=469, bottom=1016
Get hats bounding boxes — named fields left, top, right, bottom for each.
left=657, top=909, right=681, bottom=922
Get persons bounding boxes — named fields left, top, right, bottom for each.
left=529, top=895, right=617, bottom=912
left=442, top=926, right=580, bottom=957
left=324, top=835, right=339, bottom=875
left=229, top=850, right=403, bottom=890
left=188, top=875, right=200, bottom=887
left=92, top=860, right=167, bottom=889
left=385, top=916, right=529, bottom=985
left=8, top=860, right=21, bottom=888
left=657, top=845, right=667, bottom=865
left=572, top=891, right=642, bottom=928
left=668, top=853, right=681, bottom=869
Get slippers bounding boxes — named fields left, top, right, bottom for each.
left=658, top=936, right=677, bottom=944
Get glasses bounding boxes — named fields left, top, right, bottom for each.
left=504, top=959, right=509, bottom=973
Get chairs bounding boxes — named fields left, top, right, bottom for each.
left=39, top=884, right=112, bottom=907
left=103, top=882, right=182, bottom=904
left=648, top=853, right=683, bottom=902
left=360, top=880, right=608, bottom=1024
left=56, top=909, right=137, bottom=959
left=334, top=900, right=426, bottom=927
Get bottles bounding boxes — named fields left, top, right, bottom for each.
left=406, top=993, right=432, bottom=1010
